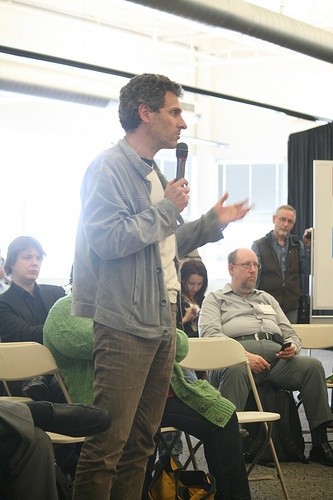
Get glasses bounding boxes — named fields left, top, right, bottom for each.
left=233, top=262, right=262, bottom=270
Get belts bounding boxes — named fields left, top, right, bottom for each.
left=234, top=331, right=273, bottom=341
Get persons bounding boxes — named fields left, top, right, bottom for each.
left=0, top=235, right=251, bottom=500
left=251, top=205, right=314, bottom=325
left=198, top=248, right=333, bottom=467
left=71, top=73, right=254, bottom=500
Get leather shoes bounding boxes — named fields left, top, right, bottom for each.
left=310, top=443, right=333, bottom=467
left=32, top=400, right=112, bottom=438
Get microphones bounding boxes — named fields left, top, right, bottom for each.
left=176, top=143, right=189, bottom=182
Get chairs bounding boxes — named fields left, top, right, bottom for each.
left=177, top=337, right=289, bottom=500
left=291, top=324, right=333, bottom=433
left=0, top=342, right=85, bottom=443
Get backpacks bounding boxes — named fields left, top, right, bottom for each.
left=242, top=390, right=309, bottom=467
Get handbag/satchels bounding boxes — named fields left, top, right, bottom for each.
left=150, top=455, right=216, bottom=500
left=298, top=293, right=310, bottom=324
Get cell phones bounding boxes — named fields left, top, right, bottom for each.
left=282, top=342, right=291, bottom=351
